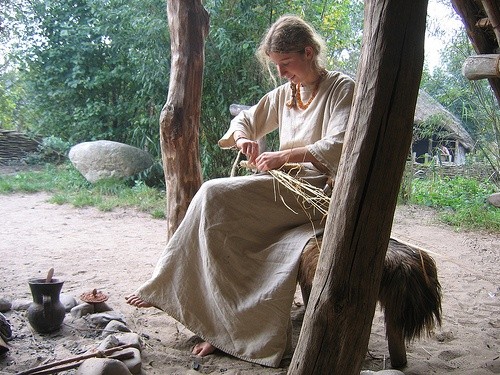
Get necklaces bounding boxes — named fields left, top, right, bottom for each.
left=292, top=67, right=326, bottom=110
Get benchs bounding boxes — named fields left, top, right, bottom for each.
left=298, top=235, right=445, bottom=369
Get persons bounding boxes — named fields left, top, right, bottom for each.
left=436, top=139, right=451, bottom=162
left=124, top=14, right=358, bottom=357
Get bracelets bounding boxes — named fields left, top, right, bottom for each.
left=234, top=135, right=249, bottom=144
